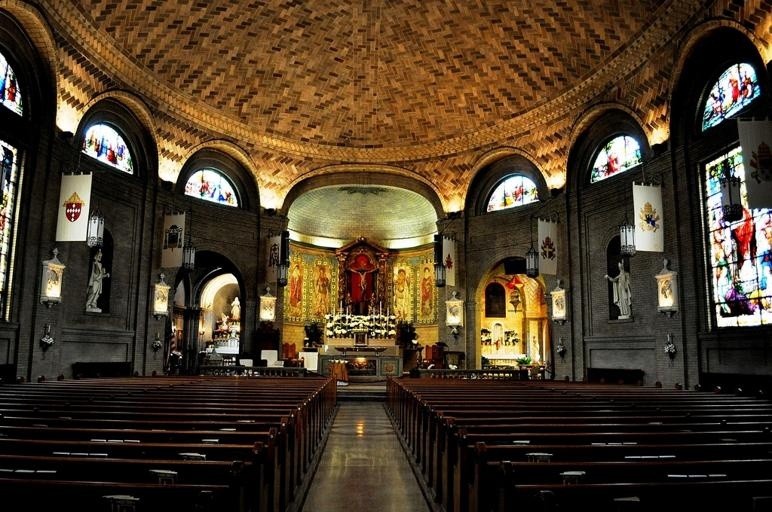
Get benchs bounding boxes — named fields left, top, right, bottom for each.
left=0, top=370, right=334, bottom=512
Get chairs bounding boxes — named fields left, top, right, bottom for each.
left=379, top=372, right=772, bottom=512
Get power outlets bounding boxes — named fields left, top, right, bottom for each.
left=180, top=193, right=196, bottom=272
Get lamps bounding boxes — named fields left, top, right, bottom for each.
left=86, top=174, right=104, bottom=249
left=38, top=248, right=66, bottom=308
left=434, top=217, right=447, bottom=287
left=655, top=258, right=679, bottom=319
left=524, top=198, right=554, bottom=279
left=277, top=231, right=288, bottom=287
left=151, top=272, right=170, bottom=319
left=444, top=291, right=464, bottom=336
left=549, top=279, right=569, bottom=326
left=714, top=111, right=747, bottom=223
left=257, top=287, right=278, bottom=323
left=618, top=180, right=636, bottom=258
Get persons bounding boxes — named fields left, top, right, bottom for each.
left=316, top=267, right=331, bottom=314
left=292, top=265, right=301, bottom=306
left=604, top=261, right=632, bottom=317
left=86, top=249, right=110, bottom=308
left=394, top=269, right=410, bottom=318
left=421, top=267, right=434, bottom=319
